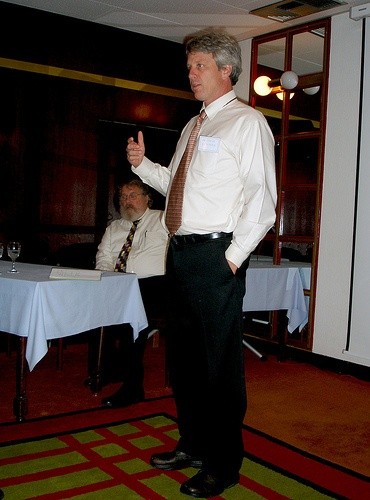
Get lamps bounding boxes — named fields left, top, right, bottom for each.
left=302, top=85, right=320, bottom=96
left=275, top=91, right=295, bottom=102
left=253, top=70, right=298, bottom=97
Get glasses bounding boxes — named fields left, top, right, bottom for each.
left=120, top=193, right=144, bottom=200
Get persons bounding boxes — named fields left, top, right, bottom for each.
left=84, top=182, right=169, bottom=406
left=125, top=26, right=277, bottom=497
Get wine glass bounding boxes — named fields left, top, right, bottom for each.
left=0, top=243, right=4, bottom=275
left=7, top=241, right=21, bottom=274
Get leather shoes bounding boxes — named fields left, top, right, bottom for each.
left=84, top=375, right=118, bottom=389
left=103, top=384, right=143, bottom=406
left=149, top=447, right=205, bottom=469
left=180, top=469, right=240, bottom=498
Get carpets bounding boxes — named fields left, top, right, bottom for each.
left=0, top=395, right=370, bottom=500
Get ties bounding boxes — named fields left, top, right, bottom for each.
left=114, top=219, right=142, bottom=273
left=164, top=96, right=238, bottom=235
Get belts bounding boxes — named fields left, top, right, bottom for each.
left=171, top=231, right=233, bottom=245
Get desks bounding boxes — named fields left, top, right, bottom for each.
left=277, top=262, right=312, bottom=343
left=0, top=259, right=150, bottom=424
left=249, top=254, right=290, bottom=262
left=240, top=263, right=307, bottom=362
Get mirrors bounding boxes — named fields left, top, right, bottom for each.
left=247, top=14, right=333, bottom=352
